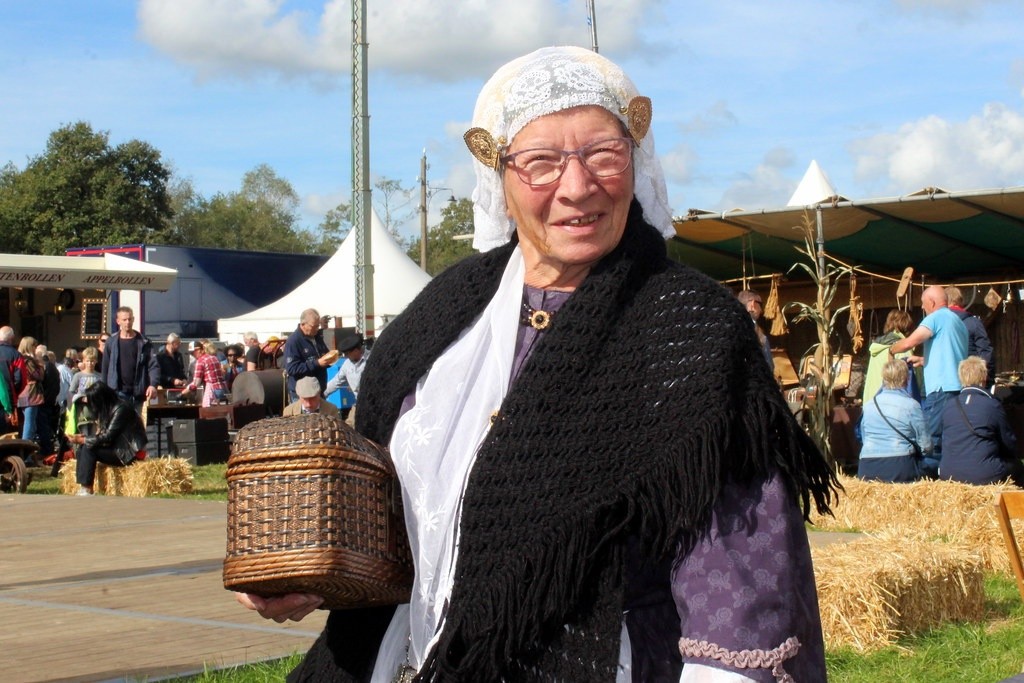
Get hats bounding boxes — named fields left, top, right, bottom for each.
left=337, top=335, right=362, bottom=352
left=184, top=341, right=204, bottom=354
left=295, top=376, right=320, bottom=398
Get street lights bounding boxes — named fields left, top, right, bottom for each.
left=414, top=147, right=460, bottom=272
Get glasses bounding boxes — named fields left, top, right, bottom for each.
left=228, top=353, right=238, bottom=357
left=100, top=339, right=106, bottom=343
left=749, top=300, right=763, bottom=309
left=306, top=324, right=320, bottom=330
left=500, top=137, right=635, bottom=187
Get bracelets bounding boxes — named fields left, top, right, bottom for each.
left=889, top=348, right=893, bottom=356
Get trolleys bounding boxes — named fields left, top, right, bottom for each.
left=0, top=435, right=40, bottom=494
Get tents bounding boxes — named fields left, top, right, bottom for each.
left=216, top=207, right=433, bottom=353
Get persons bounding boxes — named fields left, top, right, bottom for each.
left=282, top=307, right=334, bottom=402
left=234, top=45, right=846, bottom=683
left=0, top=327, right=111, bottom=476
left=283, top=376, right=339, bottom=418
left=325, top=333, right=371, bottom=428
left=65, top=382, right=148, bottom=496
left=176, top=332, right=283, bottom=408
left=737, top=289, right=774, bottom=373
left=155, top=333, right=187, bottom=390
left=101, top=307, right=160, bottom=418
left=857, top=285, right=1024, bottom=487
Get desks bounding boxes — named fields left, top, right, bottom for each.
left=147, top=403, right=201, bottom=458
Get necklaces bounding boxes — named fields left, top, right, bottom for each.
left=519, top=298, right=556, bottom=329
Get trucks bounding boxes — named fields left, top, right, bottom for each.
left=65, top=243, right=333, bottom=382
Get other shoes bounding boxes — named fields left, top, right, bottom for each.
left=76, top=489, right=95, bottom=496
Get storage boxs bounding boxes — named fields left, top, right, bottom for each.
left=172, top=442, right=231, bottom=467
left=171, top=417, right=229, bottom=444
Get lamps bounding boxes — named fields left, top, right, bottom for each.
left=14, top=290, right=27, bottom=311
left=53, top=290, right=66, bottom=322
